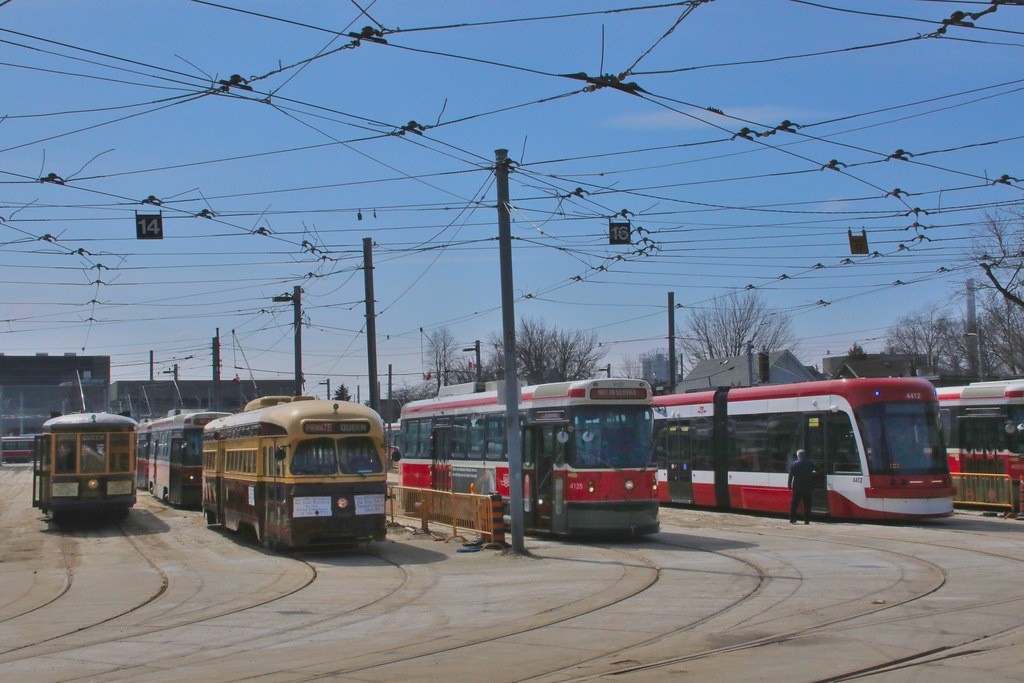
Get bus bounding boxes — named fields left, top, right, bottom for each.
left=136, top=407, right=234, bottom=508
left=31, top=368, right=140, bottom=521
left=395, top=378, right=662, bottom=542
left=384, top=418, right=400, bottom=471
left=657, top=375, right=955, bottom=523
left=200, top=394, right=402, bottom=555
left=936, top=378, right=1024, bottom=511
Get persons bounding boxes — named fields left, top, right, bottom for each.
left=788, top=450, right=822, bottom=525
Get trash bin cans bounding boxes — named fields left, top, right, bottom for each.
left=1005, top=479, right=1020, bottom=514
